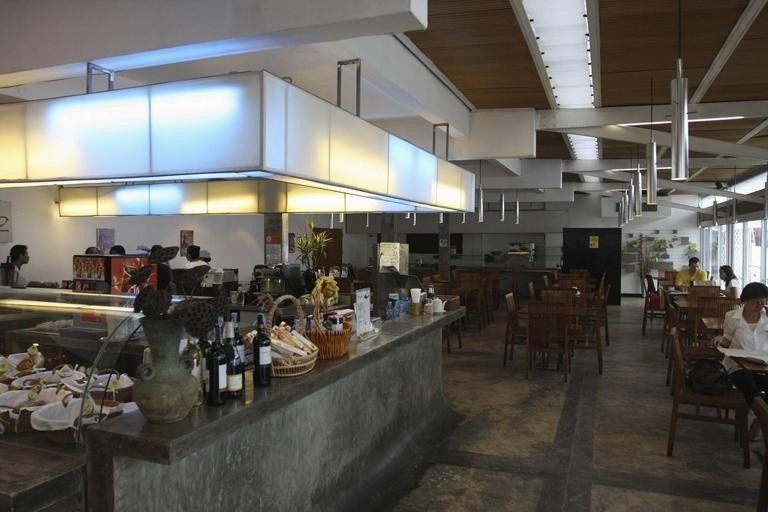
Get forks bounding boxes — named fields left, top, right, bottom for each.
left=11, top=370, right=85, bottom=389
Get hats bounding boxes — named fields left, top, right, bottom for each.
left=199, top=249, right=211, bottom=260
left=136, top=243, right=149, bottom=248
left=148, top=245, right=168, bottom=263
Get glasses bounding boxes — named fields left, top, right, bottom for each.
left=749, top=298, right=763, bottom=305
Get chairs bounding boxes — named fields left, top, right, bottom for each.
left=502, top=291, right=537, bottom=371
left=666, top=303, right=725, bottom=397
left=560, top=279, right=585, bottom=288
left=574, top=283, right=612, bottom=350
left=540, top=288, right=577, bottom=358
left=496, top=270, right=521, bottom=312
left=751, top=396, right=768, bottom=512
left=665, top=270, right=679, bottom=281
left=525, top=302, right=572, bottom=383
left=451, top=272, right=481, bottom=320
left=421, top=277, right=438, bottom=295
left=553, top=269, right=562, bottom=281
left=661, top=303, right=691, bottom=359
left=666, top=326, right=751, bottom=469
left=564, top=295, right=605, bottom=376
left=641, top=274, right=667, bottom=335
left=542, top=274, right=551, bottom=290
left=591, top=271, right=607, bottom=299
left=433, top=274, right=449, bottom=295
left=526, top=281, right=536, bottom=299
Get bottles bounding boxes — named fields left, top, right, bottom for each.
left=196, top=335, right=212, bottom=400
left=230, top=312, right=246, bottom=389
left=252, top=315, right=272, bottom=387
left=327, top=315, right=343, bottom=331
left=223, top=322, right=243, bottom=399
left=206, top=324, right=227, bottom=407
left=428, top=285, right=434, bottom=301
left=179, top=339, right=203, bottom=406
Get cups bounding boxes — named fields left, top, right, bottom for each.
left=410, top=288, right=421, bottom=303
left=572, top=287, right=577, bottom=294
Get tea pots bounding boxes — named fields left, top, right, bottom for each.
left=432, top=297, right=447, bottom=312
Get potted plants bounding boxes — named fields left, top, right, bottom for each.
left=123, top=244, right=230, bottom=423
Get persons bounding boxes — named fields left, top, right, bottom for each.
left=130, top=244, right=148, bottom=254
left=9, top=245, right=32, bottom=286
left=183, top=246, right=209, bottom=270
left=718, top=266, right=742, bottom=301
left=675, top=257, right=708, bottom=290
left=198, top=250, right=211, bottom=266
left=709, top=282, right=768, bottom=442
left=149, top=245, right=169, bottom=271
left=108, top=244, right=124, bottom=254
left=84, top=246, right=99, bottom=255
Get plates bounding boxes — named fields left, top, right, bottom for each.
left=434, top=310, right=446, bottom=315
left=61, top=373, right=135, bottom=393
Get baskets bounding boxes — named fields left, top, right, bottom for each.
left=0, top=382, right=80, bottom=434
left=292, top=280, right=352, bottom=360
left=31, top=392, right=122, bottom=444
left=10, top=364, right=88, bottom=389
left=90, top=368, right=134, bottom=402
left=433, top=294, right=461, bottom=311
left=243, top=295, right=319, bottom=379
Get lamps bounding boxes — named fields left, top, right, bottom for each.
left=477, top=159, right=484, bottom=223
left=620, top=191, right=624, bottom=227
left=646, top=77, right=657, bottom=205
left=764, top=161, right=768, bottom=220
left=498, top=188, right=505, bottom=222
left=628, top=171, right=635, bottom=221
left=438, top=212, right=444, bottom=224
left=697, top=193, right=700, bottom=227
left=618, top=202, right=622, bottom=228
left=624, top=182, right=629, bottom=224
left=460, top=212, right=466, bottom=224
left=339, top=212, right=344, bottom=223
left=732, top=165, right=736, bottom=224
left=412, top=212, right=417, bottom=226
left=713, top=193, right=717, bottom=225
left=365, top=212, right=369, bottom=227
left=329, top=212, right=334, bottom=229
left=635, top=143, right=642, bottom=217
left=513, top=188, right=520, bottom=225
left=670, top=0, right=689, bottom=181
left=404, top=212, right=410, bottom=219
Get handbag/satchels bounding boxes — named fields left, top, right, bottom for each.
left=650, top=292, right=659, bottom=309
left=687, top=358, right=730, bottom=398
